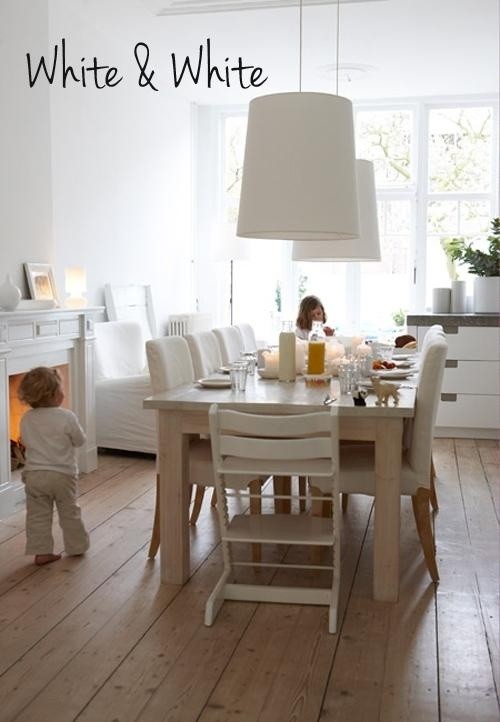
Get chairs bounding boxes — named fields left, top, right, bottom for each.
left=93, top=318, right=160, bottom=455
left=141, top=321, right=449, bottom=634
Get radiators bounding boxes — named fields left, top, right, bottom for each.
left=167, top=313, right=192, bottom=336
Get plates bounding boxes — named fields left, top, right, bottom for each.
left=391, top=355, right=409, bottom=360
left=197, top=378, right=231, bottom=389
left=217, top=366, right=230, bottom=373
left=389, top=361, right=416, bottom=368
left=357, top=381, right=403, bottom=390
left=258, top=369, right=280, bottom=379
left=369, top=368, right=420, bottom=381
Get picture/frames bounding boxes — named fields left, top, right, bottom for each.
left=23, top=261, right=59, bottom=308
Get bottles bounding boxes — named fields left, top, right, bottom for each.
left=278, top=320, right=297, bottom=384
left=240, top=351, right=257, bottom=376
left=306, top=320, right=326, bottom=385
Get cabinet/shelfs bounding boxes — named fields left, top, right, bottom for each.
left=406, top=310, right=500, bottom=441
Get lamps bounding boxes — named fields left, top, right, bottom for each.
left=290, top=0, right=383, bottom=261
left=214, top=231, right=254, bottom=325
left=64, top=265, right=88, bottom=309
left=235, top=0, right=362, bottom=244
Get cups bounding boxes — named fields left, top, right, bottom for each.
left=467, top=296, right=473, bottom=313
left=227, top=361, right=249, bottom=392
left=450, top=280, right=467, bottom=314
left=337, top=364, right=351, bottom=395
left=344, top=345, right=362, bottom=385
left=431, top=287, right=450, bottom=313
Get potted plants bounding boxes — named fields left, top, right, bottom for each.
left=443, top=217, right=500, bottom=313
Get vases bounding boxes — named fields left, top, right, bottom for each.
left=0, top=272, right=22, bottom=312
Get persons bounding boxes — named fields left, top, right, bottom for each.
left=18, top=367, right=91, bottom=567
left=294, top=295, right=335, bottom=342
